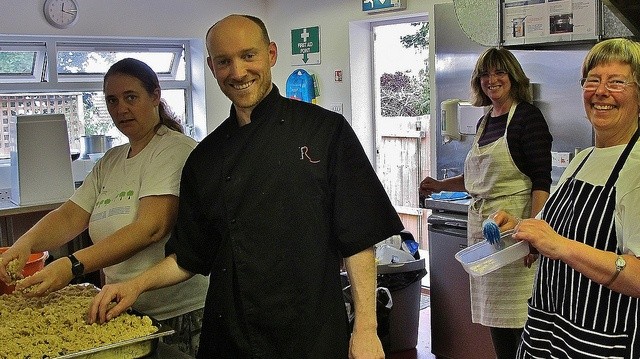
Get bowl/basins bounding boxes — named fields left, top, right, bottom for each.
left=86, top=153, right=106, bottom=160
left=0, top=245, right=50, bottom=294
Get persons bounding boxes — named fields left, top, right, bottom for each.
left=86, top=14, right=405, bottom=359
left=419, top=49, right=553, bottom=359
left=0, top=58, right=211, bottom=358
left=488, top=35, right=640, bottom=359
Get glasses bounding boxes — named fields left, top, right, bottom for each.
left=578, top=78, right=640, bottom=92
left=480, top=71, right=510, bottom=78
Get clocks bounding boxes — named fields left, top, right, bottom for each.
left=44, top=0, right=80, bottom=30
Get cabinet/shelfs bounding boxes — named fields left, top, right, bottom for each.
left=1, top=211, right=71, bottom=261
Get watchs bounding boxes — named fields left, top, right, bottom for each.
left=603, top=255, right=626, bottom=288
left=67, top=255, right=84, bottom=278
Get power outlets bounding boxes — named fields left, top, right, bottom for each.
left=557, top=153, right=571, bottom=168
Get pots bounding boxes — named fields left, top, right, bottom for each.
left=81, top=135, right=106, bottom=159
left=106, top=135, right=116, bottom=153
